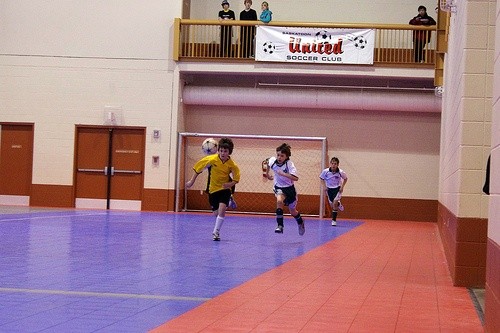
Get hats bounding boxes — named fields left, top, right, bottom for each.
left=221, top=0, right=229, bottom=4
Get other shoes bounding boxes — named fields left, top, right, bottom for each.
left=249, top=54, right=255, bottom=58
left=419, top=59, right=426, bottom=63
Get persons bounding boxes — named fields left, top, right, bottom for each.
left=265, top=143, right=305, bottom=235
left=409, top=5, right=437, bottom=64
left=257, top=1, right=273, bottom=23
left=218, top=0, right=236, bottom=57
left=184, top=137, right=240, bottom=241
left=319, top=157, right=348, bottom=226
left=240, top=0, right=257, bottom=58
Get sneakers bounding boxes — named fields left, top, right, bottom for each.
left=332, top=220, right=337, bottom=226
left=298, top=221, right=305, bottom=235
left=213, top=231, right=220, bottom=241
left=338, top=201, right=344, bottom=211
left=229, top=195, right=237, bottom=208
left=275, top=225, right=284, bottom=233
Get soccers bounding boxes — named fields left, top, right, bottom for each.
left=354, top=35, right=367, bottom=49
left=262, top=41, right=275, bottom=55
left=201, top=137, right=219, bottom=155
left=314, top=30, right=331, bottom=43
